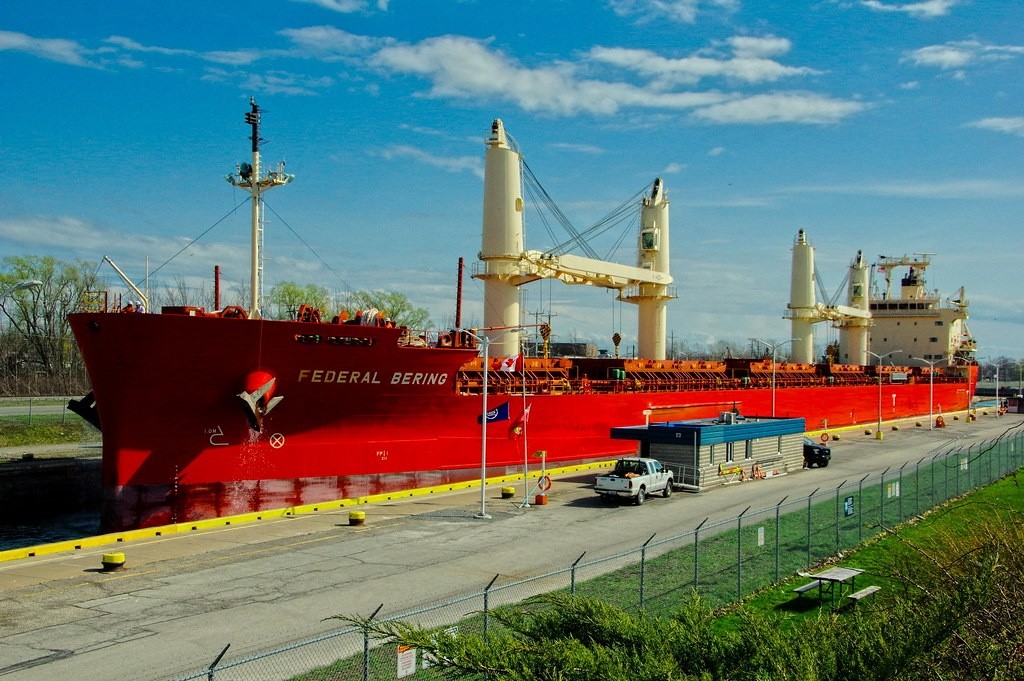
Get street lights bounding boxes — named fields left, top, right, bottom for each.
left=445, top=319, right=526, bottom=522
left=1008, top=360, right=1024, bottom=396
left=863, top=348, right=902, bottom=442
left=910, top=355, right=951, bottom=430
left=953, top=356, right=989, bottom=423
left=749, top=336, right=802, bottom=418
left=986, top=359, right=1010, bottom=418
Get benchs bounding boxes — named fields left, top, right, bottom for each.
left=792, top=580, right=828, bottom=606
left=847, top=585, right=882, bottom=613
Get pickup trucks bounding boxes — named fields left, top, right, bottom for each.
left=593, top=456, right=674, bottom=506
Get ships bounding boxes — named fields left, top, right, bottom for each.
left=61, top=249, right=983, bottom=537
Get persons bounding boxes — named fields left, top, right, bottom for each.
left=385, top=318, right=392, bottom=328
left=121, top=300, right=146, bottom=313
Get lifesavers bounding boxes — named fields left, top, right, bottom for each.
left=937, top=416, right=943, bottom=423
left=538, top=476, right=552, bottom=491
left=820, top=433, right=830, bottom=441
left=439, top=333, right=452, bottom=346
left=541, top=382, right=548, bottom=392
left=972, top=409, right=977, bottom=415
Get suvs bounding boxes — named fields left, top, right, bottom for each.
left=804, top=437, right=831, bottom=468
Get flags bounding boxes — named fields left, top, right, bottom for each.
left=878, top=267, right=886, bottom=272
left=478, top=401, right=510, bottom=424
left=491, top=352, right=523, bottom=372
left=509, top=404, right=531, bottom=440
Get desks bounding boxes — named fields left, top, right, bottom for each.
left=810, top=566, right=866, bottom=605
